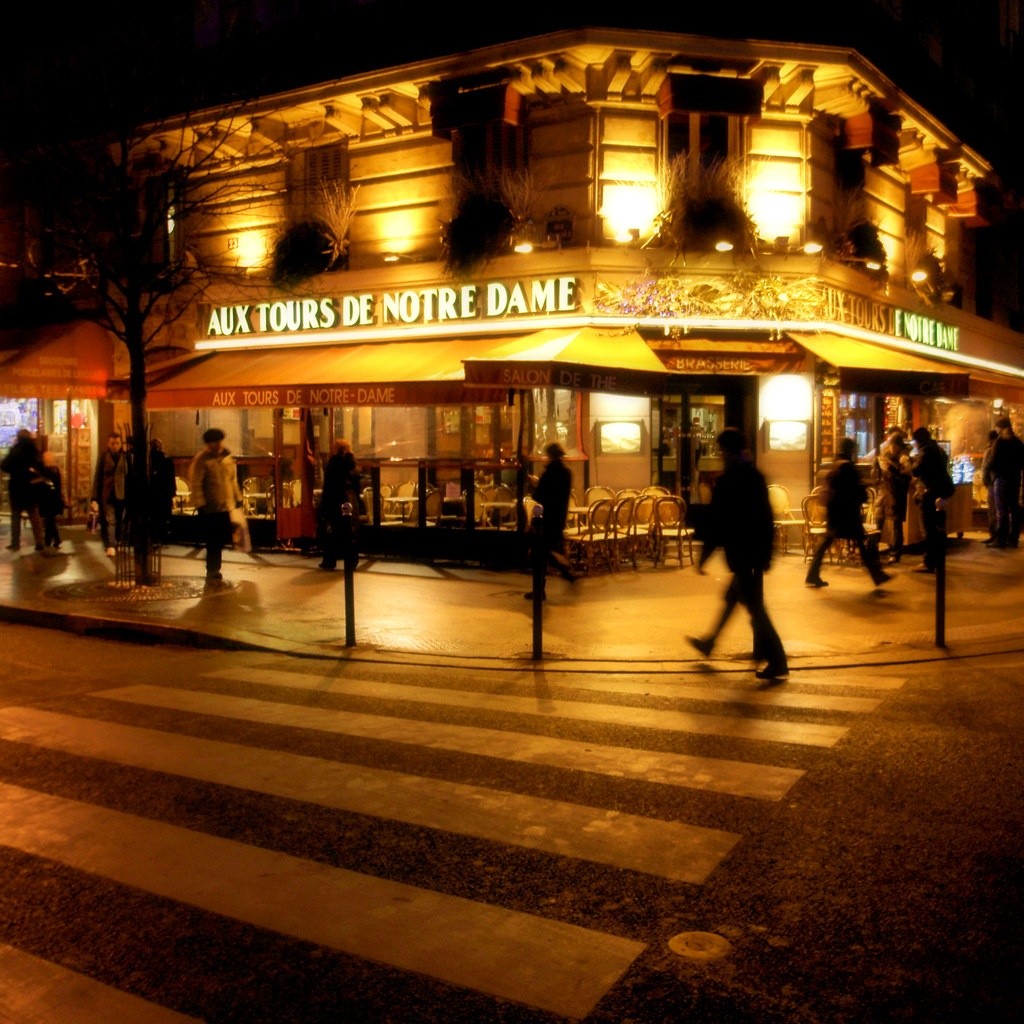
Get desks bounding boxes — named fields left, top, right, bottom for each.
left=567, top=506, right=595, bottom=571
left=384, top=496, right=419, bottom=522
left=176, top=491, right=192, bottom=516
left=243, top=493, right=271, bottom=513
left=481, top=501, right=515, bottom=530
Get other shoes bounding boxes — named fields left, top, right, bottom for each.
left=981, top=537, right=1018, bottom=548
left=207, top=572, right=222, bottom=579
left=35, top=543, right=44, bottom=551
left=806, top=577, right=829, bottom=587
left=756, top=663, right=788, bottom=678
left=912, top=567, right=935, bottom=574
left=688, top=637, right=713, bottom=657
left=106, top=547, right=116, bottom=557
left=886, top=556, right=901, bottom=566
left=875, top=573, right=894, bottom=586
left=318, top=562, right=337, bottom=570
left=524, top=590, right=546, bottom=601
left=738, top=653, right=768, bottom=661
left=6, top=545, right=20, bottom=550
left=54, top=540, right=62, bottom=547
left=566, top=573, right=577, bottom=592
left=44, top=540, right=52, bottom=546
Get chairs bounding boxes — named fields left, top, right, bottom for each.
left=170, top=468, right=883, bottom=579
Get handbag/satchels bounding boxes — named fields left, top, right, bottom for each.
left=230, top=506, right=252, bottom=553
left=86, top=499, right=98, bottom=530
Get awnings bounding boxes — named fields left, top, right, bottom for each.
left=959, top=367, right=1024, bottom=400
left=0, top=323, right=970, bottom=412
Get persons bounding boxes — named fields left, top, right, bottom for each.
left=686, top=429, right=789, bottom=678
left=806, top=438, right=898, bottom=587
left=188, top=428, right=243, bottom=578
left=524, top=442, right=572, bottom=600
left=91, top=433, right=175, bottom=556
left=981, top=416, right=1024, bottom=548
left=0, top=430, right=63, bottom=550
left=657, top=419, right=707, bottom=503
left=266, top=458, right=295, bottom=493
left=862, top=425, right=955, bottom=572
left=314, top=441, right=367, bottom=570
